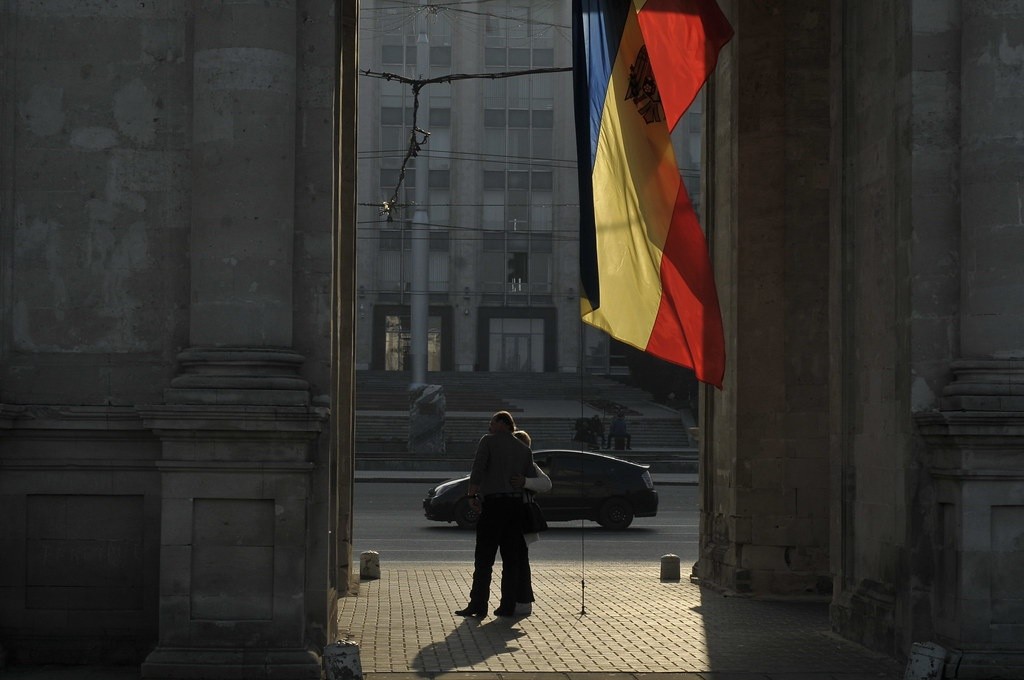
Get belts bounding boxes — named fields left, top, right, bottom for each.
left=490, top=493, right=522, bottom=499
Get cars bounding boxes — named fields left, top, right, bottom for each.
left=423, top=449, right=659, bottom=530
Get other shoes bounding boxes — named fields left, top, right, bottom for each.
left=494, top=604, right=516, bottom=615
left=515, top=602, right=531, bottom=614
left=455, top=603, right=488, bottom=615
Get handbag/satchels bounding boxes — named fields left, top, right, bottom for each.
left=523, top=503, right=548, bottom=534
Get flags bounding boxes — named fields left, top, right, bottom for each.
left=572, top=0, right=734, bottom=392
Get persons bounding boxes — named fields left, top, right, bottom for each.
left=455, top=411, right=532, bottom=618
left=513, top=431, right=553, bottom=614
left=574, top=416, right=600, bottom=450
left=606, top=411, right=633, bottom=450
left=590, top=414, right=606, bottom=448
